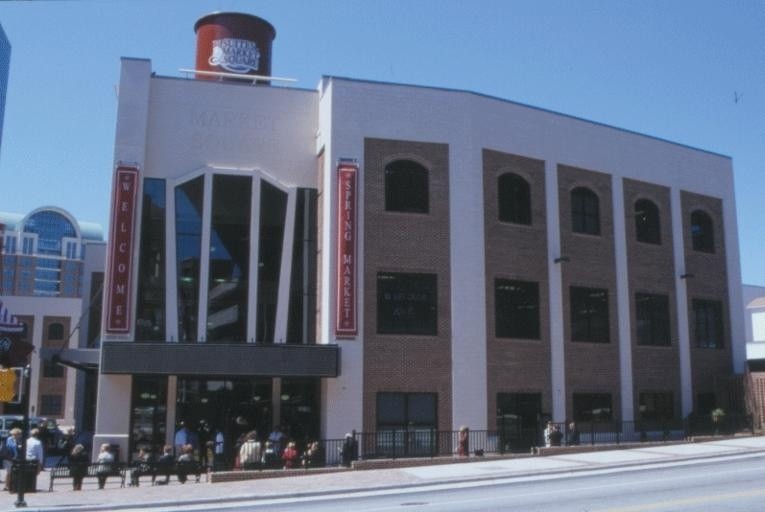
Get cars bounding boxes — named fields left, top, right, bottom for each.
left=494, top=413, right=522, bottom=432
left=0, top=430, right=68, bottom=462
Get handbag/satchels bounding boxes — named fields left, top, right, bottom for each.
left=0, top=444, right=15, bottom=460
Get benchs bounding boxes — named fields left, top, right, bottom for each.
left=48, top=459, right=200, bottom=493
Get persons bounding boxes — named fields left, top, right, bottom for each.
left=544, top=421, right=555, bottom=447
left=549, top=425, right=563, bottom=446
left=456, top=426, right=466, bottom=455
left=233, top=430, right=319, bottom=471
left=565, top=422, right=580, bottom=445
left=1, top=417, right=225, bottom=490
left=339, top=433, right=359, bottom=467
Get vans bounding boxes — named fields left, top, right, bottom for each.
left=574, top=408, right=614, bottom=423
left=0, top=414, right=61, bottom=439
